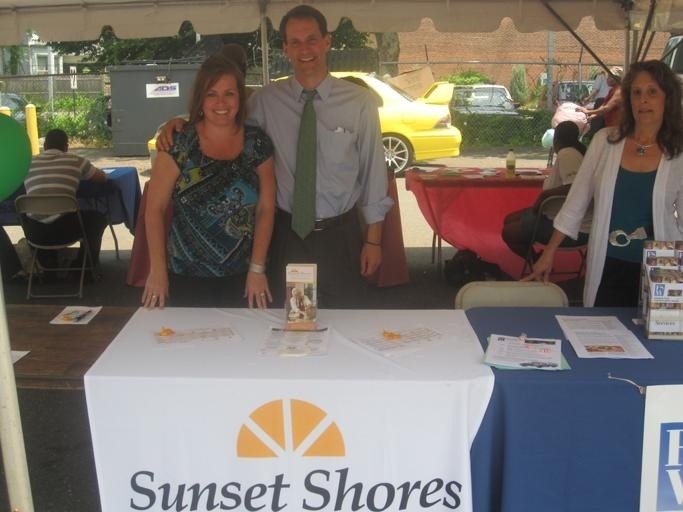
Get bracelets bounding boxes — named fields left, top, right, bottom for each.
left=248, top=264, right=267, bottom=275
left=365, top=241, right=382, bottom=247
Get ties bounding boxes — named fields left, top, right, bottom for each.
left=288, top=88, right=322, bottom=242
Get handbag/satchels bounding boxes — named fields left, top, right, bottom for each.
left=443, top=248, right=502, bottom=286
left=124, top=177, right=175, bottom=289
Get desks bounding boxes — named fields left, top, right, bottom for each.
left=397, top=165, right=545, bottom=281
left=2, top=305, right=682, bottom=511
left=122, top=169, right=410, bottom=291
left=0, top=165, right=143, bottom=264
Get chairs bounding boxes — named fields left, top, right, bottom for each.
left=11, top=192, right=102, bottom=305
left=450, top=279, right=570, bottom=308
left=519, top=188, right=593, bottom=288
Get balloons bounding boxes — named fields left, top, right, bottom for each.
left=0, top=113, right=31, bottom=201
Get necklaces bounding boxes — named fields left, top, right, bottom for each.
left=629, top=136, right=660, bottom=155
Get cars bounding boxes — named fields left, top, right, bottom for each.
left=1, top=94, right=30, bottom=128
left=446, top=80, right=595, bottom=119
left=146, top=73, right=461, bottom=175
left=659, top=33, right=682, bottom=80
left=85, top=94, right=112, bottom=135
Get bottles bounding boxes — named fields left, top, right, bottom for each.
left=505, top=148, right=516, bottom=179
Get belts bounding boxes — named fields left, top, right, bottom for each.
left=274, top=204, right=360, bottom=232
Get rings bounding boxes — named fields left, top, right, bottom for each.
left=261, top=292, right=267, bottom=296
left=152, top=295, right=157, bottom=298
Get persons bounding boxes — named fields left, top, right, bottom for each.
left=25, top=129, right=106, bottom=284
left=156, top=7, right=392, bottom=309
left=290, top=288, right=312, bottom=319
left=139, top=55, right=276, bottom=310
left=518, top=62, right=682, bottom=306
left=220, top=43, right=255, bottom=101
left=577, top=66, right=628, bottom=140
left=502, top=120, right=594, bottom=265
left=0, top=226, right=38, bottom=282
